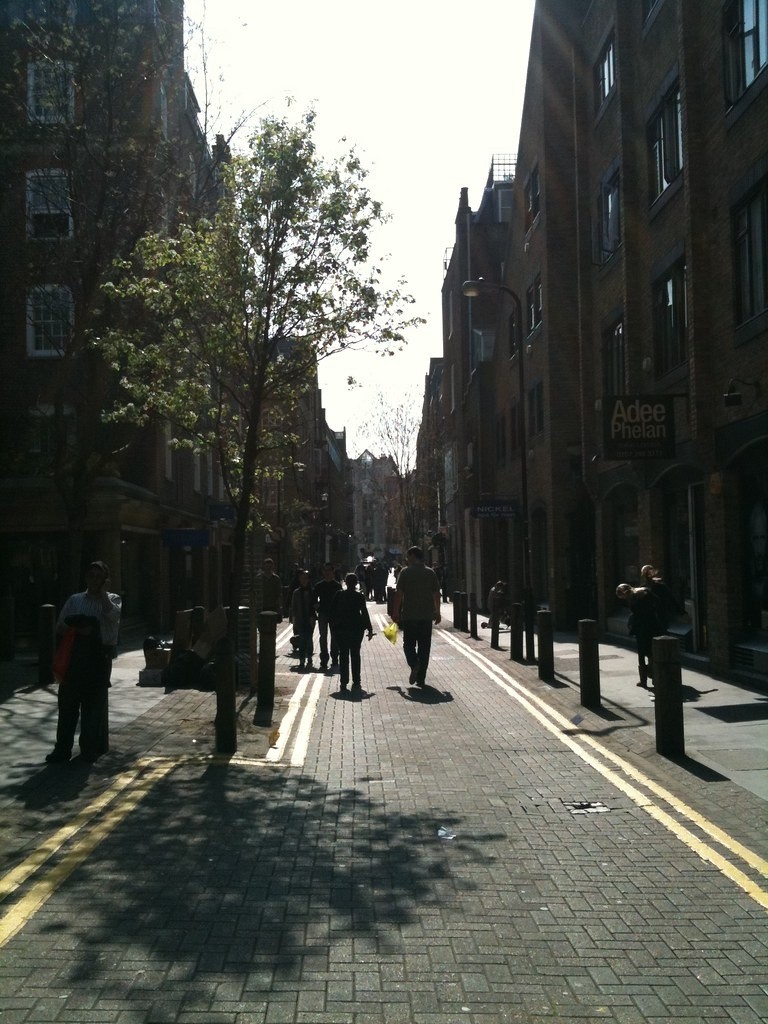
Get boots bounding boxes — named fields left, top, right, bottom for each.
left=637, top=665, right=648, bottom=687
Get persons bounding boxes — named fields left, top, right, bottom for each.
left=481, top=581, right=504, bottom=630
left=254, top=558, right=372, bottom=693
left=289, top=557, right=448, bottom=604
left=616, top=564, right=688, bottom=688
left=45, top=561, right=121, bottom=767
left=391, top=546, right=442, bottom=687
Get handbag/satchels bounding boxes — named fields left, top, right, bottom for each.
left=382, top=622, right=399, bottom=645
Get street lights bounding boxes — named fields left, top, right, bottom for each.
left=276, top=464, right=306, bottom=576
left=419, top=482, right=441, bottom=533
left=460, top=277, right=538, bottom=664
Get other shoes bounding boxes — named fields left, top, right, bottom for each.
left=340, top=684, right=346, bottom=691
left=80, top=747, right=97, bottom=761
left=418, top=681, right=425, bottom=686
left=319, top=666, right=328, bottom=672
left=351, top=686, right=361, bottom=694
left=46, top=749, right=72, bottom=762
left=328, top=666, right=338, bottom=672
left=409, top=663, right=422, bottom=685
